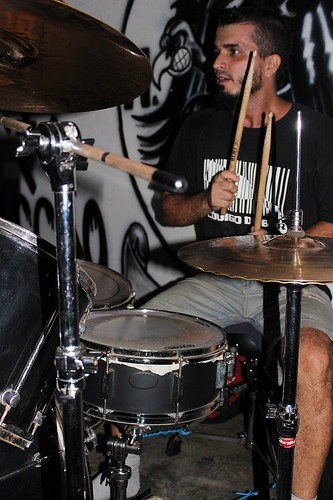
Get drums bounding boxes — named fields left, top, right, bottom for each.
left=74, top=257, right=135, bottom=310
left=79, top=306, right=233, bottom=429
left=0, top=389, right=86, bottom=500
left=0, top=218, right=98, bottom=458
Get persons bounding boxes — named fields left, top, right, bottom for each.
left=78, top=6, right=333, bottom=500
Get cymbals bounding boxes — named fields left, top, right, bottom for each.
left=0, top=0, right=154, bottom=113
left=181, top=233, right=333, bottom=286
